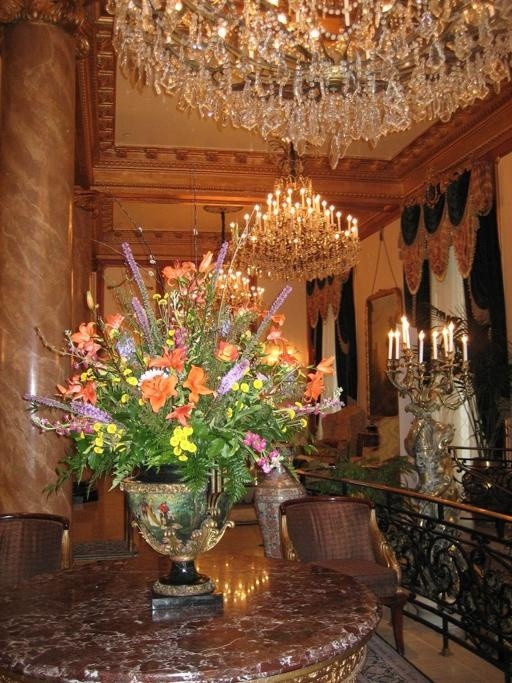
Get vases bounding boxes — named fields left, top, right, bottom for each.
left=118, top=457, right=306, bottom=601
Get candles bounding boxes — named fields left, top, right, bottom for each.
left=385, top=315, right=470, bottom=362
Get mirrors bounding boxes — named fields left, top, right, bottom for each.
left=364, top=286, right=404, bottom=420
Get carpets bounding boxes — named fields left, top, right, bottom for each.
left=355, top=631, right=435, bottom=683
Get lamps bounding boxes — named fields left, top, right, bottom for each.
left=104, top=0, right=511, bottom=171
left=228, top=135, right=361, bottom=280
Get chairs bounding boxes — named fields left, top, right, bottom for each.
left=1, top=511, right=73, bottom=590
left=278, top=497, right=415, bottom=655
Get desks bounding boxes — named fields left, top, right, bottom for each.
left=1, top=555, right=382, bottom=683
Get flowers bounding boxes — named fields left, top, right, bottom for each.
left=24, top=239, right=347, bottom=512
